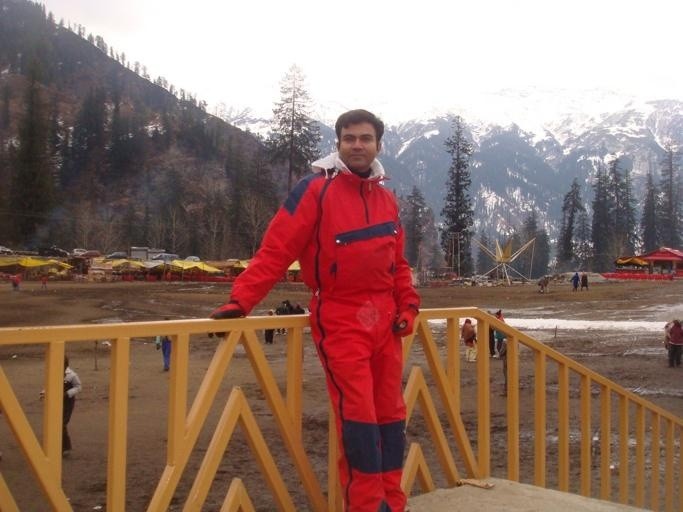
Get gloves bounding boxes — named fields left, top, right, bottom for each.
left=156, top=343, right=160, bottom=351
left=394, top=307, right=418, bottom=337
left=208, top=301, right=242, bottom=337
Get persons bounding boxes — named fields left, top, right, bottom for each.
left=668, top=319, right=683, bottom=368
left=155, top=317, right=172, bottom=371
left=488, top=309, right=521, bottom=397
left=39, top=355, right=82, bottom=455
left=581, top=270, right=588, bottom=292
left=264, top=299, right=305, bottom=344
left=570, top=272, right=580, bottom=292
left=208, top=109, right=421, bottom=512
left=538, top=277, right=545, bottom=292
left=461, top=319, right=477, bottom=362
left=41, top=272, right=48, bottom=288
left=15, top=273, right=21, bottom=290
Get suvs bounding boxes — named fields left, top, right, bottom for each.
left=72, top=249, right=87, bottom=256
left=106, top=252, right=127, bottom=259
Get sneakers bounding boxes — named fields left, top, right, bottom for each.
left=466, top=353, right=497, bottom=361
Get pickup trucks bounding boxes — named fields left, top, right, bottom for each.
left=39, top=247, right=70, bottom=257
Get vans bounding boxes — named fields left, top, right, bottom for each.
left=83, top=251, right=100, bottom=257
left=152, top=254, right=178, bottom=260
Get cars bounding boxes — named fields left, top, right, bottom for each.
left=0, top=246, right=13, bottom=255
left=185, top=256, right=200, bottom=262
